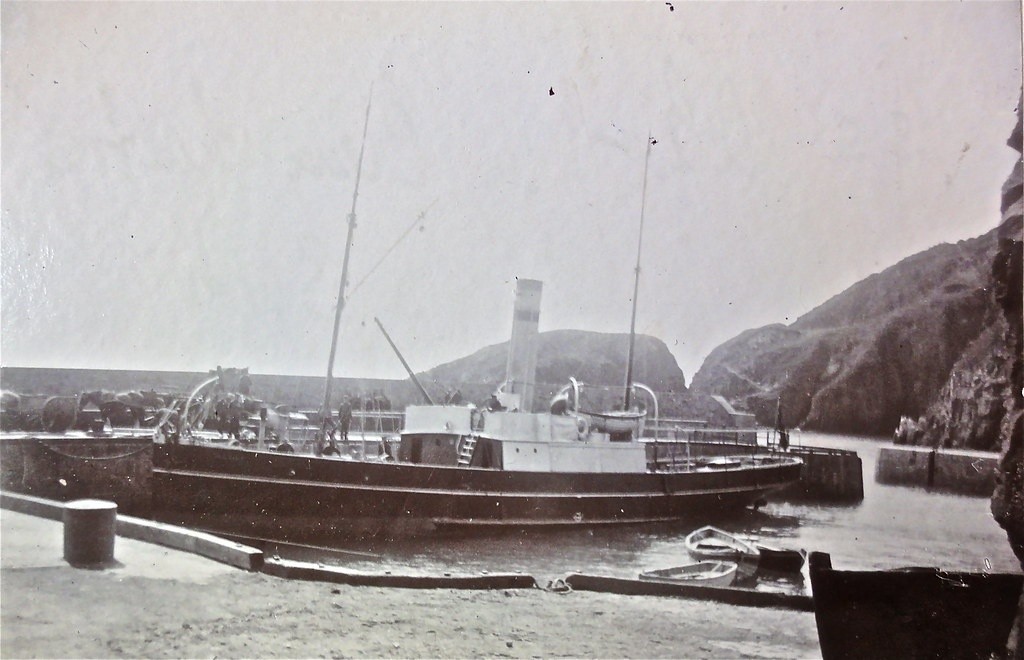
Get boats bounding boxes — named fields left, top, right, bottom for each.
left=574, top=410, right=647, bottom=436
left=684, top=525, right=760, bottom=579
left=637, top=560, right=739, bottom=589
left=149, top=87, right=803, bottom=534
left=746, top=538, right=808, bottom=575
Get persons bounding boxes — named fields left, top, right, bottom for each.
left=443, top=388, right=463, bottom=405
left=551, top=399, right=570, bottom=416
left=215, top=392, right=241, bottom=439
left=339, top=395, right=352, bottom=439
left=277, top=437, right=295, bottom=454
left=322, top=440, right=341, bottom=456
left=378, top=436, right=392, bottom=457
left=489, top=395, right=501, bottom=411
left=317, top=402, right=337, bottom=429
